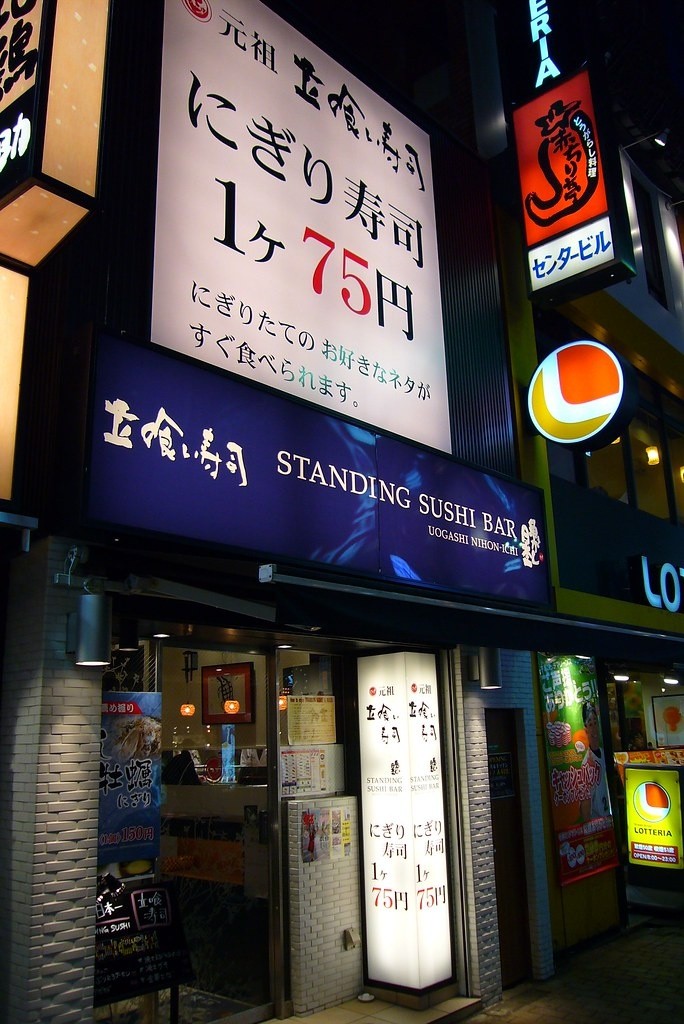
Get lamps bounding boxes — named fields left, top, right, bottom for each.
left=180, top=701, right=196, bottom=719
left=223, top=699, right=240, bottom=715
left=279, top=695, right=287, bottom=711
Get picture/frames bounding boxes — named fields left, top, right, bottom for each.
left=201, top=661, right=256, bottom=725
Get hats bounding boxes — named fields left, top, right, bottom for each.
left=582, top=701, right=597, bottom=722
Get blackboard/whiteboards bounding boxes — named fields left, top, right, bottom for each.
left=89, top=881, right=196, bottom=1008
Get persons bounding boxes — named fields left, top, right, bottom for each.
left=629, top=731, right=645, bottom=751
left=308, top=829, right=316, bottom=862
left=581, top=702, right=610, bottom=821
